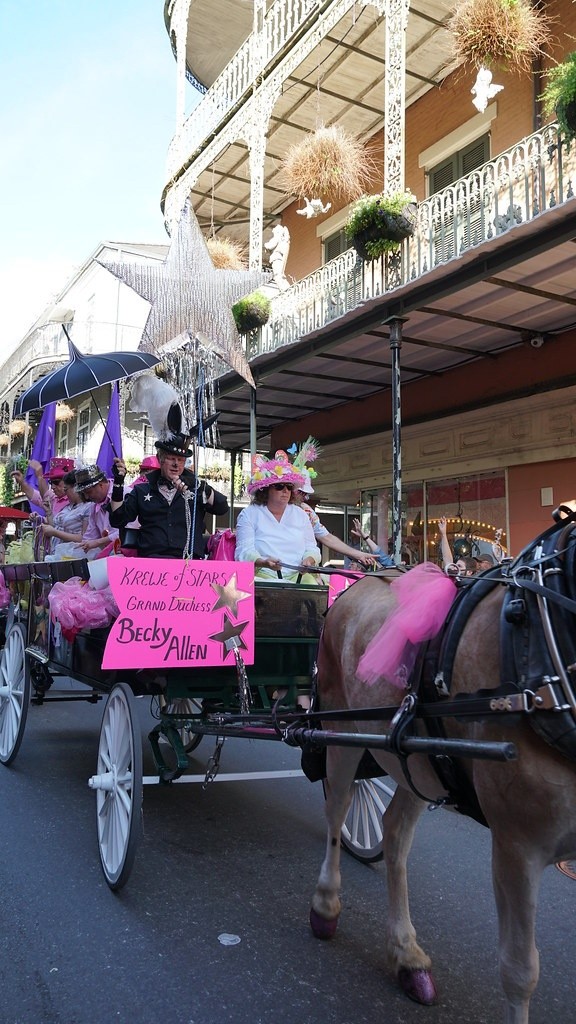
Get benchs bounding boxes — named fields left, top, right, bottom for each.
left=118, top=526, right=214, bottom=554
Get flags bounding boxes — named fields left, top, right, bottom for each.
left=95, top=382, right=122, bottom=480
left=25, top=403, right=56, bottom=517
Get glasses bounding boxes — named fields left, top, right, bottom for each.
left=48, top=478, right=63, bottom=485
left=63, top=486, right=75, bottom=492
left=297, top=489, right=309, bottom=497
left=271, top=484, right=293, bottom=490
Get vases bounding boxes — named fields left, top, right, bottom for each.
left=351, top=202, right=419, bottom=261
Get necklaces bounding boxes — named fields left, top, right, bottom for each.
left=268, top=506, right=283, bottom=514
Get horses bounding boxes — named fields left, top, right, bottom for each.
left=306, top=512, right=576, bottom=1024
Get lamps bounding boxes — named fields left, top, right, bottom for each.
left=438, top=479, right=481, bottom=561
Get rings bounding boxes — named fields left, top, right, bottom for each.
left=181, top=482, right=185, bottom=485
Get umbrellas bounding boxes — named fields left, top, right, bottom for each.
left=15, top=324, right=163, bottom=458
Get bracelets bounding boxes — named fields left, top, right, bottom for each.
left=363, top=536, right=370, bottom=540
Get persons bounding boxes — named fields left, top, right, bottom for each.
left=235, top=439, right=322, bottom=717
left=8, top=456, right=120, bottom=560
left=438, top=516, right=493, bottom=577
left=129, top=456, right=160, bottom=489
left=265, top=225, right=288, bottom=292
left=350, top=518, right=410, bottom=571
left=473, top=68, right=492, bottom=114
left=299, top=490, right=381, bottom=566
left=109, top=400, right=228, bottom=693
left=296, top=197, right=331, bottom=218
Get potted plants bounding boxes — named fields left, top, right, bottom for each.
left=530, top=55, right=576, bottom=148
left=231, top=291, right=273, bottom=336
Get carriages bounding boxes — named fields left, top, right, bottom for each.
left=0, top=498, right=576, bottom=1024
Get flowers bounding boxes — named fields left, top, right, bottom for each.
left=247, top=460, right=305, bottom=495
left=340, top=187, right=417, bottom=260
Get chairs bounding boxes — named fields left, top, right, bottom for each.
left=207, top=533, right=237, bottom=561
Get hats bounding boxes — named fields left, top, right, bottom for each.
left=42, top=458, right=76, bottom=479
left=128, top=374, right=220, bottom=457
left=244, top=450, right=305, bottom=495
left=287, top=434, right=324, bottom=494
left=75, top=465, right=107, bottom=493
left=138, top=456, right=162, bottom=469
left=472, top=554, right=494, bottom=564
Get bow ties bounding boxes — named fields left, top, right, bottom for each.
left=158, top=477, right=174, bottom=489
